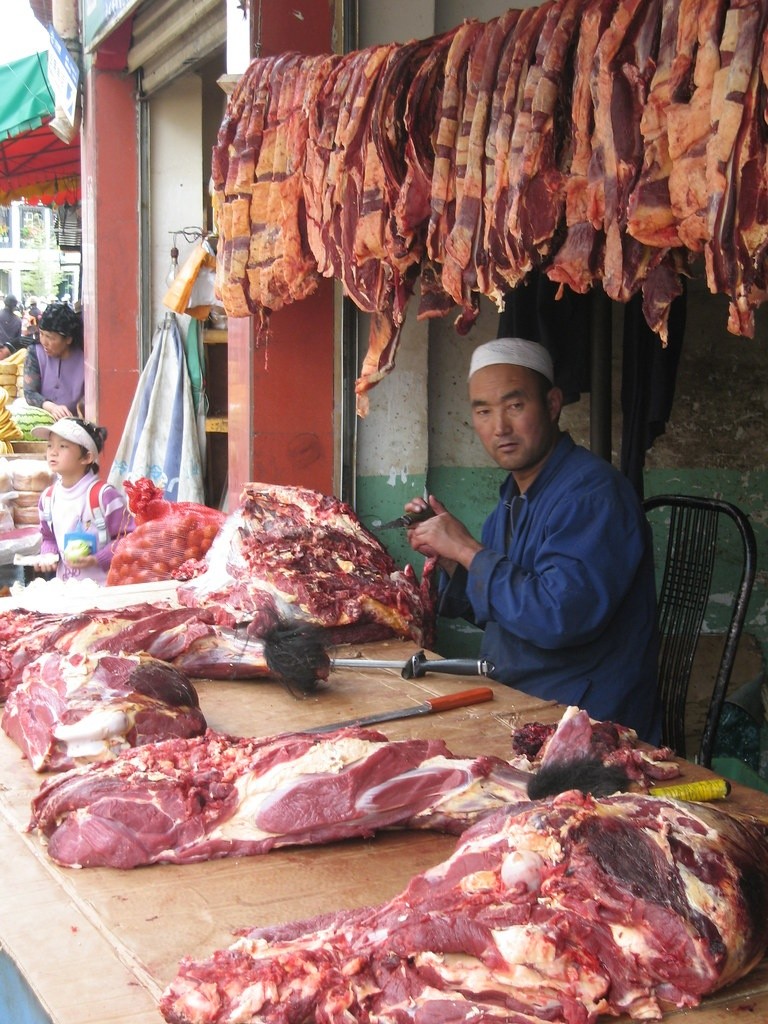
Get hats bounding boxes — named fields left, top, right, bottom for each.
left=30, top=417, right=99, bottom=465
left=38, top=303, right=80, bottom=332
left=4, top=294, right=17, bottom=307
left=468, top=337, right=553, bottom=381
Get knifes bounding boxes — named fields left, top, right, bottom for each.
left=295, top=687, right=495, bottom=736
left=375, top=501, right=445, bottom=533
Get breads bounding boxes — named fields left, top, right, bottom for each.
left=0, top=362, right=24, bottom=402
left=0, top=472, right=51, bottom=525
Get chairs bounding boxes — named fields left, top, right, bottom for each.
left=637, top=493, right=757, bottom=769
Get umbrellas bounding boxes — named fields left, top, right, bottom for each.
left=108, top=312, right=204, bottom=504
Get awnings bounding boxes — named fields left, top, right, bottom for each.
left=0, top=51, right=80, bottom=206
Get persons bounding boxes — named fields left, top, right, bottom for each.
left=405, top=339, right=663, bottom=747
left=0, top=295, right=81, bottom=357
left=23, top=304, right=85, bottom=419
left=31, top=417, right=137, bottom=588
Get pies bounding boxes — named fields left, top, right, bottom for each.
left=0, top=388, right=22, bottom=454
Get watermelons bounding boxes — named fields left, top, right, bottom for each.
left=11, top=406, right=55, bottom=443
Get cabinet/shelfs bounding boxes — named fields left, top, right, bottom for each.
left=200, top=327, right=229, bottom=434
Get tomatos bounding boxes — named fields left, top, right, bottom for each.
left=106, top=510, right=219, bottom=585
